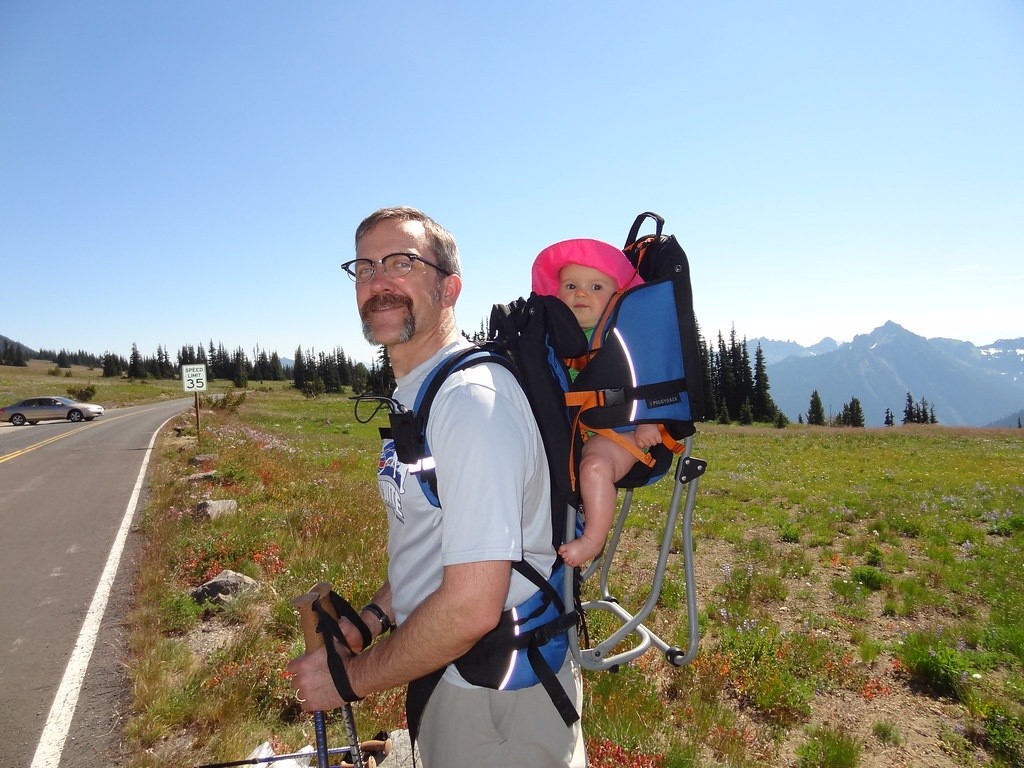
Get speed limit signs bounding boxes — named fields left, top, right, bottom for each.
left=181, top=364, right=207, bottom=392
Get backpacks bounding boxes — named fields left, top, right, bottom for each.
left=476, top=294, right=594, bottom=575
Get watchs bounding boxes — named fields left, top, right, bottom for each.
left=361, top=603, right=391, bottom=636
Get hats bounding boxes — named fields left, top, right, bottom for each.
left=534, top=237, right=646, bottom=299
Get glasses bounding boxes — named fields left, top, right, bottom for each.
left=340, top=251, right=450, bottom=284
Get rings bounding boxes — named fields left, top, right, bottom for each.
left=295, top=688, right=306, bottom=703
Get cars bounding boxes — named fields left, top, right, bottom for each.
left=0, top=396, right=105, bottom=426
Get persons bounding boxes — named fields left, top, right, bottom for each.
left=286, top=206, right=596, bottom=768
left=551, top=252, right=675, bottom=570
